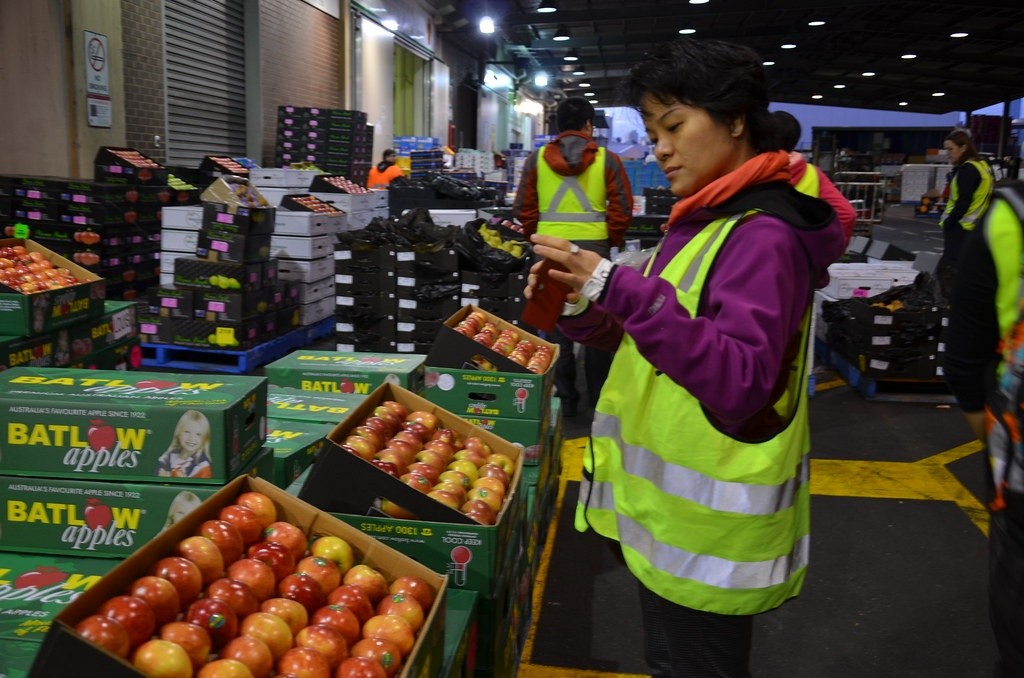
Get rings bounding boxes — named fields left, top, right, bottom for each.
left=569, top=244, right=579, bottom=253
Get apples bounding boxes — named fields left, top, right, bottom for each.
left=112, top=150, right=159, bottom=167
left=71, top=492, right=433, bottom=678
left=235, top=184, right=266, bottom=208
left=451, top=311, right=551, bottom=374
left=0, top=245, right=93, bottom=295
left=291, top=196, right=337, bottom=213
left=339, top=398, right=514, bottom=526
left=478, top=225, right=522, bottom=257
left=209, top=156, right=247, bottom=172
left=322, top=176, right=368, bottom=194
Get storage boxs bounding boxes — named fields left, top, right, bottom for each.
left=0, top=105, right=1024, bottom=678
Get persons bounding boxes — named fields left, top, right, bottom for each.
left=934, top=130, right=1024, bottom=678
left=152, top=491, right=202, bottom=539
left=148, top=410, right=212, bottom=478
left=367, top=149, right=404, bottom=188
left=513, top=97, right=634, bottom=416
left=56, top=330, right=69, bottom=364
left=523, top=41, right=841, bottom=678
left=771, top=110, right=856, bottom=256
left=32, top=295, right=50, bottom=333
